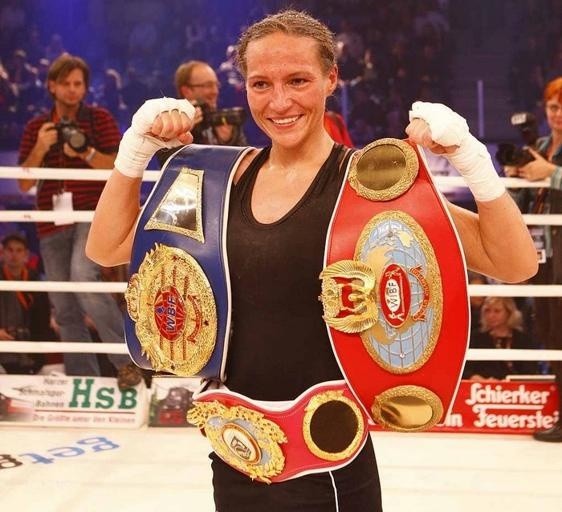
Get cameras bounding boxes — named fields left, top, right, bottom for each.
left=494, top=112, right=541, bottom=167
left=189, top=97, right=245, bottom=129
left=54, top=113, right=88, bottom=154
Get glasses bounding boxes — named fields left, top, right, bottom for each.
left=188, top=82, right=221, bottom=88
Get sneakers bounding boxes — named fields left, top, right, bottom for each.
left=119, top=367, right=139, bottom=386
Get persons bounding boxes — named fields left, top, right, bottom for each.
left=2, top=0, right=561, bottom=442
left=85, top=11, right=539, bottom=512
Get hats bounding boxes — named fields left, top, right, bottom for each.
left=2, top=233, right=26, bottom=246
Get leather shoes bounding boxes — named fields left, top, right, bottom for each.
left=534, top=425, right=562, bottom=442
